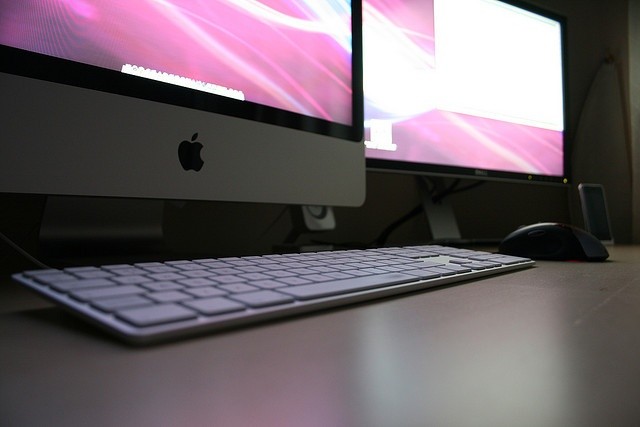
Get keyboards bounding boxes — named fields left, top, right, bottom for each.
left=12, top=244, right=535, bottom=345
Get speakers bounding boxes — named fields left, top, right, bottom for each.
left=567, top=183, right=615, bottom=246
left=290, top=203, right=337, bottom=232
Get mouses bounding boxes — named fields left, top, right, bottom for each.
left=499, top=222, right=609, bottom=262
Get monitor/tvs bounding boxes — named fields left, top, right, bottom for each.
left=363, top=0, right=574, bottom=253
left=1, top=1, right=367, bottom=268
